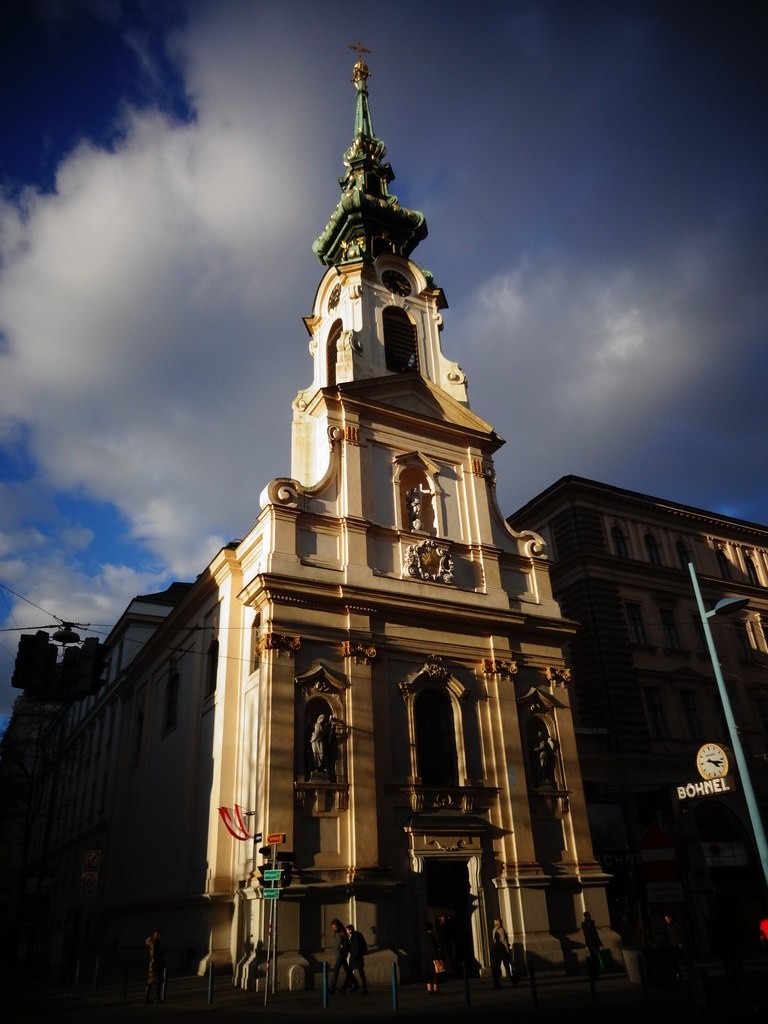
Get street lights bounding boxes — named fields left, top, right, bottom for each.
left=688, top=561, right=768, bottom=884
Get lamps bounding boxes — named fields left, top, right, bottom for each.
left=696, top=742, right=735, bottom=780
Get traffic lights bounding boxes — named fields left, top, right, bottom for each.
left=10, top=629, right=108, bottom=706
left=257, top=845, right=272, bottom=887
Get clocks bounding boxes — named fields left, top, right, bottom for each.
left=381, top=269, right=412, bottom=297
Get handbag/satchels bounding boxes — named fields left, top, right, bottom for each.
left=491, top=933, right=506, bottom=960
left=354, top=933, right=367, bottom=955
left=433, top=959, right=446, bottom=973
left=597, top=945, right=615, bottom=970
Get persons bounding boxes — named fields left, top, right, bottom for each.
left=143, top=928, right=169, bottom=1005
left=419, top=922, right=445, bottom=996
left=533, top=728, right=558, bottom=782
left=325, top=917, right=361, bottom=995
left=490, top=917, right=521, bottom=987
left=661, top=913, right=690, bottom=979
left=338, top=924, right=370, bottom=996
left=581, top=910, right=606, bottom=980
left=312, top=713, right=333, bottom=774
left=405, top=485, right=427, bottom=531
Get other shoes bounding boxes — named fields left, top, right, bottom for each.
left=337, top=989, right=347, bottom=994
left=143, top=998, right=152, bottom=1003
left=427, top=990, right=440, bottom=995
left=350, top=987, right=360, bottom=992
left=154, top=998, right=166, bottom=1005
